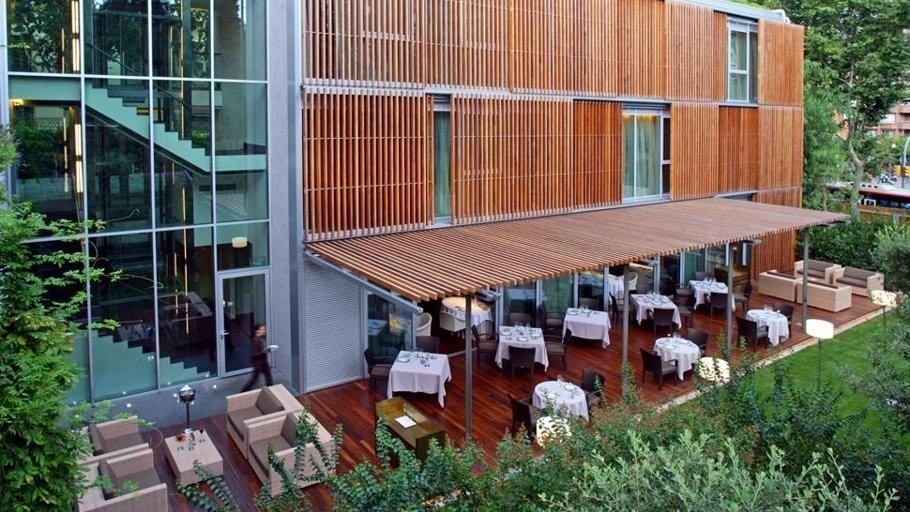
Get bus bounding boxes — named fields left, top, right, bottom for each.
left=818, top=180, right=910, bottom=209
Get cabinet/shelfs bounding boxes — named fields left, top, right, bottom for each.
left=714, top=262, right=751, bottom=291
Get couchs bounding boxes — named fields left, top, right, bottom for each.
left=796, top=276, right=853, bottom=312
left=71, top=450, right=169, bottom=511
left=248, top=411, right=338, bottom=499
left=831, top=267, right=884, bottom=296
left=793, top=260, right=841, bottom=284
left=72, top=414, right=150, bottom=464
left=756, top=269, right=807, bottom=302
left=225, top=384, right=302, bottom=462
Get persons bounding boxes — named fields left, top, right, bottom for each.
left=240, top=324, right=273, bottom=393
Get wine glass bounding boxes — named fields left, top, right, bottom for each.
left=199, top=424, right=205, bottom=443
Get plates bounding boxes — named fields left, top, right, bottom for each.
left=567, top=310, right=591, bottom=315
left=397, top=352, right=429, bottom=363
left=662, top=338, right=689, bottom=350
left=500, top=328, right=541, bottom=341
left=544, top=383, right=581, bottom=402
left=636, top=295, right=669, bottom=305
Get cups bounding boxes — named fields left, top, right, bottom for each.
left=699, top=276, right=720, bottom=291
left=760, top=304, right=784, bottom=318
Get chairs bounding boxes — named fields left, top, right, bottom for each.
left=774, top=303, right=794, bottom=338
left=379, top=260, right=662, bottom=355
left=507, top=396, right=544, bottom=444
left=471, top=325, right=498, bottom=371
left=645, top=307, right=672, bottom=341
left=684, top=328, right=708, bottom=376
left=736, top=316, right=769, bottom=353
left=537, top=328, right=570, bottom=373
left=671, top=283, right=696, bottom=327
left=640, top=348, right=678, bottom=389
left=363, top=347, right=394, bottom=394
left=693, top=271, right=715, bottom=280
left=733, top=282, right=753, bottom=310
left=579, top=368, right=603, bottom=425
left=163, top=430, right=223, bottom=487
left=373, top=398, right=446, bottom=470
left=704, top=291, right=726, bottom=321
left=508, top=346, right=536, bottom=384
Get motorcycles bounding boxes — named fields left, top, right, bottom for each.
left=879, top=171, right=898, bottom=185
